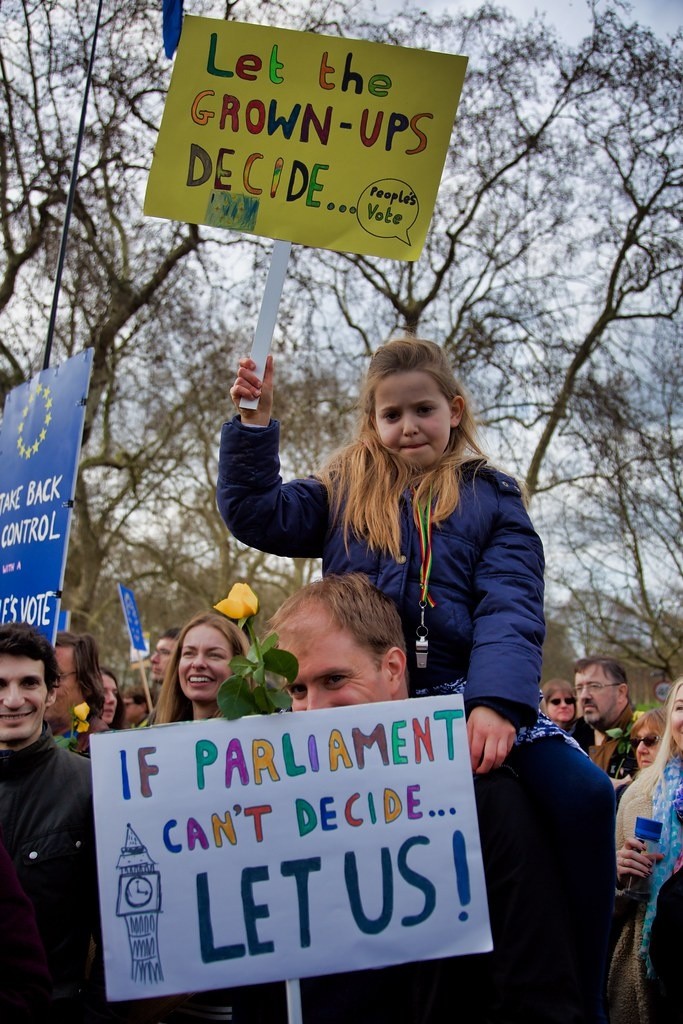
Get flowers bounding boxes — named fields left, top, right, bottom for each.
left=211, top=583, right=299, bottom=720
left=54, top=702, right=91, bottom=753
left=605, top=702, right=656, bottom=779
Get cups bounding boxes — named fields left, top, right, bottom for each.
left=624, top=816, right=663, bottom=903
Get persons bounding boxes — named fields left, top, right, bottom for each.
left=169, top=571, right=616, bottom=1024
left=0, top=623, right=128, bottom=1024
left=44, top=614, right=281, bottom=1024
left=216, top=339, right=546, bottom=774
left=540, top=656, right=683, bottom=1024
left=0, top=842, right=52, bottom=1023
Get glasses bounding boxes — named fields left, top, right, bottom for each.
left=630, top=736, right=662, bottom=749
left=57, top=671, right=76, bottom=682
left=571, top=683, right=620, bottom=696
left=549, top=697, right=574, bottom=705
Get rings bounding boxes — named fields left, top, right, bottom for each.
left=622, top=858, right=626, bottom=867
